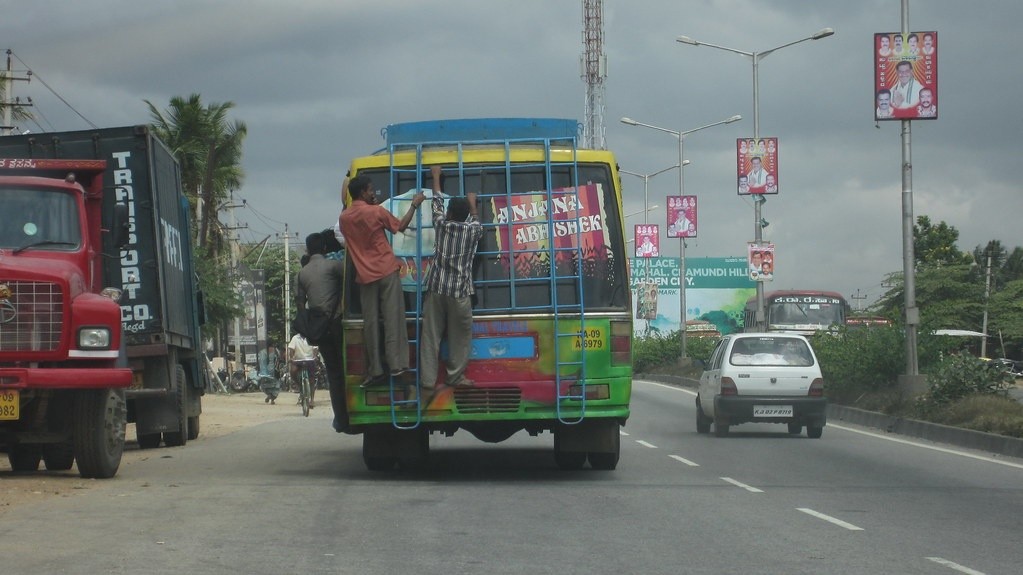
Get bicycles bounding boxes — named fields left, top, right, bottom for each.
left=287, top=358, right=320, bottom=417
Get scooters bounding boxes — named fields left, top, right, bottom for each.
left=211, top=367, right=250, bottom=393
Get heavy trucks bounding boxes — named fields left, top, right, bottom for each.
left=0, top=125, right=208, bottom=481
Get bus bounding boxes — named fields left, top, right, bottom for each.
left=341, top=114, right=634, bottom=475
left=847, top=316, right=895, bottom=335
left=743, top=290, right=848, bottom=356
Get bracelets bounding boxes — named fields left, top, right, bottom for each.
left=411, top=204, right=417, bottom=209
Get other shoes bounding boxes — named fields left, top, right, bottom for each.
left=332, top=415, right=348, bottom=427
left=454, top=378, right=475, bottom=388
left=271, top=398, right=275, bottom=404
left=310, top=401, right=315, bottom=407
left=265, top=395, right=271, bottom=402
left=359, top=375, right=385, bottom=387
left=390, top=371, right=404, bottom=376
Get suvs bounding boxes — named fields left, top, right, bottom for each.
left=693, top=331, right=827, bottom=438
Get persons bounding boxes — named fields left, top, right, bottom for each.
left=739, top=140, right=777, bottom=193
left=288, top=334, right=319, bottom=409
left=340, top=177, right=425, bottom=389
left=258, top=338, right=284, bottom=405
left=750, top=251, right=772, bottom=279
left=668, top=197, right=696, bottom=236
left=418, top=164, right=483, bottom=391
left=297, top=229, right=361, bottom=432
left=637, top=225, right=658, bottom=255
left=876, top=34, right=937, bottom=118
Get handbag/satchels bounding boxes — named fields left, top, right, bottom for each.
left=292, top=307, right=332, bottom=345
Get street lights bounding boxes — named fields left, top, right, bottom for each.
left=673, top=25, right=837, bottom=333
left=619, top=158, right=693, bottom=338
left=618, top=115, right=745, bottom=359
